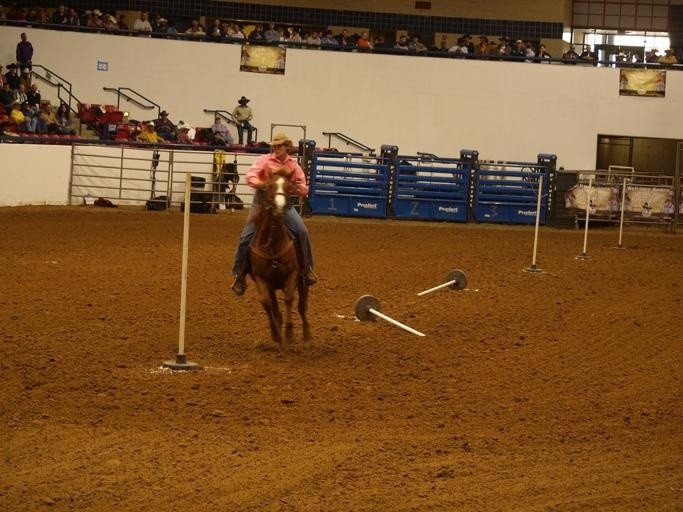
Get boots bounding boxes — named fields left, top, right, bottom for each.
left=247, top=138, right=255, bottom=144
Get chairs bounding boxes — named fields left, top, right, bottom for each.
left=0, top=64, right=251, bottom=150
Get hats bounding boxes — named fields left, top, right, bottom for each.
left=10, top=100, right=21, bottom=106
left=145, top=121, right=157, bottom=126
left=159, top=110, right=170, bottom=116
left=268, top=132, right=293, bottom=148
left=651, top=48, right=672, bottom=53
left=92, top=9, right=103, bottom=17
left=237, top=96, right=250, bottom=104
left=82, top=10, right=93, bottom=17
left=157, top=18, right=168, bottom=25
left=0, top=63, right=33, bottom=74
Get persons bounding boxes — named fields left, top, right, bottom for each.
left=616, top=48, right=676, bottom=69
left=0, top=1, right=129, bottom=36
left=231, top=134, right=318, bottom=296
left=563, top=44, right=597, bottom=65
left=393, top=34, right=552, bottom=64
left=133, top=12, right=177, bottom=37
left=0, top=63, right=257, bottom=150
left=185, top=19, right=384, bottom=54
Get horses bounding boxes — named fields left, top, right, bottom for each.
left=248, top=166, right=317, bottom=344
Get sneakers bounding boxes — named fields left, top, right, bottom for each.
left=232, top=278, right=245, bottom=296
left=305, top=271, right=317, bottom=285
